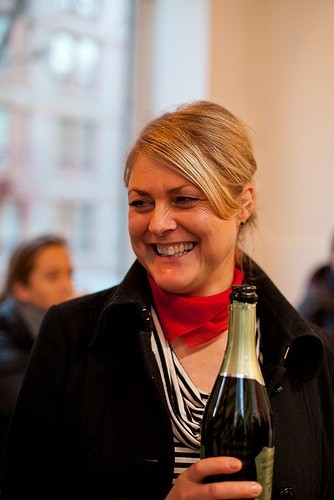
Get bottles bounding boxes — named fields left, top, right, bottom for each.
left=199, top=286, right=276, bottom=500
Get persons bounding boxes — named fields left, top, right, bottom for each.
left=1, top=235, right=76, bottom=428
left=295, top=258, right=334, bottom=346
left=4, top=101, right=334, bottom=498
